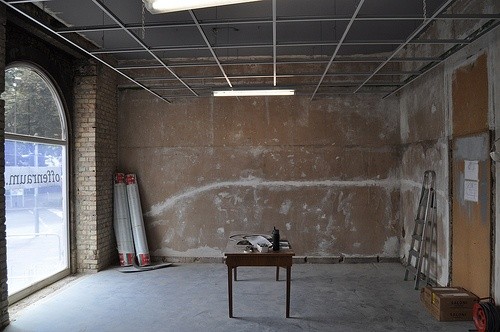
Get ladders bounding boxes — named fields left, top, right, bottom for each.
left=403, top=170, right=436, bottom=291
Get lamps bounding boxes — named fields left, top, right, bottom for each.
left=143, top=0, right=263, bottom=15
left=210, top=89, right=298, bottom=97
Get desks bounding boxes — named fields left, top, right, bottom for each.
left=223, top=231, right=296, bottom=319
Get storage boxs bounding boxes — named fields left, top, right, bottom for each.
left=422, top=285, right=481, bottom=322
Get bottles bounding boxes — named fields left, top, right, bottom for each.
left=272, top=226, right=279, bottom=251
